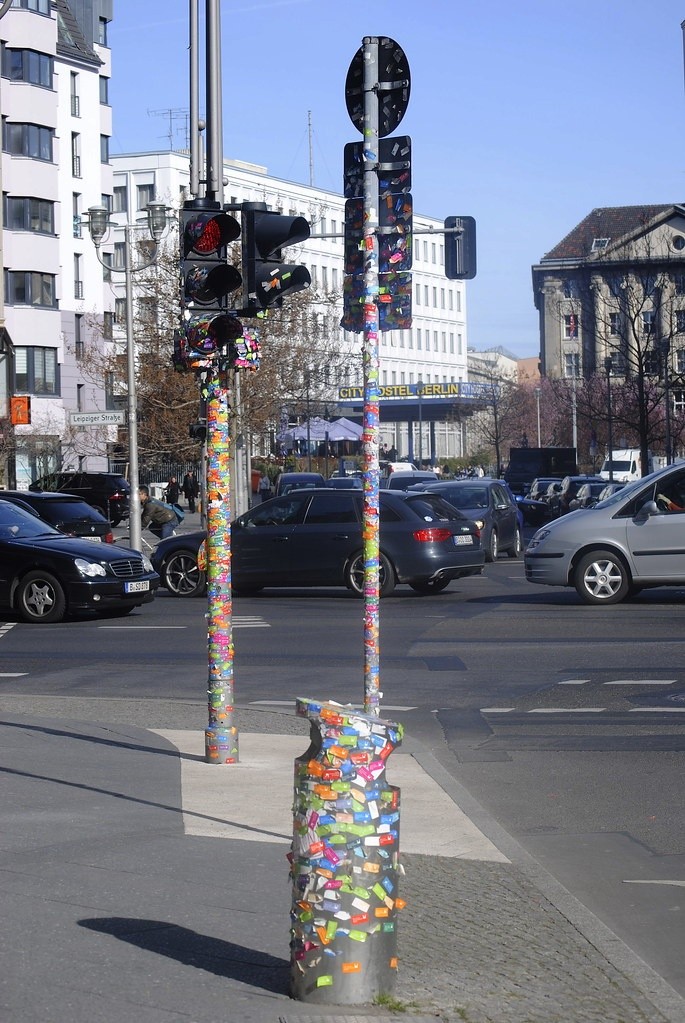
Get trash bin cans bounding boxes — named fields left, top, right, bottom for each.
left=286, top=698, right=404, bottom=1004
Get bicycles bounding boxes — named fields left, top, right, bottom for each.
left=159, top=486, right=169, bottom=503
left=112, top=524, right=176, bottom=556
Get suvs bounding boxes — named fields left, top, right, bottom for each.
left=28, top=470, right=132, bottom=528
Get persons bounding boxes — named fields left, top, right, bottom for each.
left=382, top=443, right=398, bottom=462
left=183, top=470, right=198, bottom=513
left=658, top=494, right=685, bottom=510
left=164, top=477, right=183, bottom=505
left=257, top=469, right=281, bottom=502
left=279, top=442, right=364, bottom=457
left=139, top=485, right=178, bottom=539
left=434, top=464, right=484, bottom=479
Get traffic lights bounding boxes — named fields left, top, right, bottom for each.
left=178, top=209, right=242, bottom=369
left=240, top=202, right=314, bottom=316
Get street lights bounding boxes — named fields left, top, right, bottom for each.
left=79, top=196, right=177, bottom=553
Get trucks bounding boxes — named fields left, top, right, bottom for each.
left=599, top=449, right=654, bottom=482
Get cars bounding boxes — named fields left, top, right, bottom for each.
left=521, top=472, right=635, bottom=520
left=146, top=487, right=488, bottom=598
left=0, top=499, right=160, bottom=626
left=524, top=460, right=685, bottom=606
left=270, top=459, right=548, bottom=561
left=0, top=486, right=116, bottom=546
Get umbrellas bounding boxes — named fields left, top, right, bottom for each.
left=277, top=417, right=358, bottom=440
left=331, top=417, right=363, bottom=437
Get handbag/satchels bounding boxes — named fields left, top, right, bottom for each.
left=169, top=504, right=186, bottom=525
